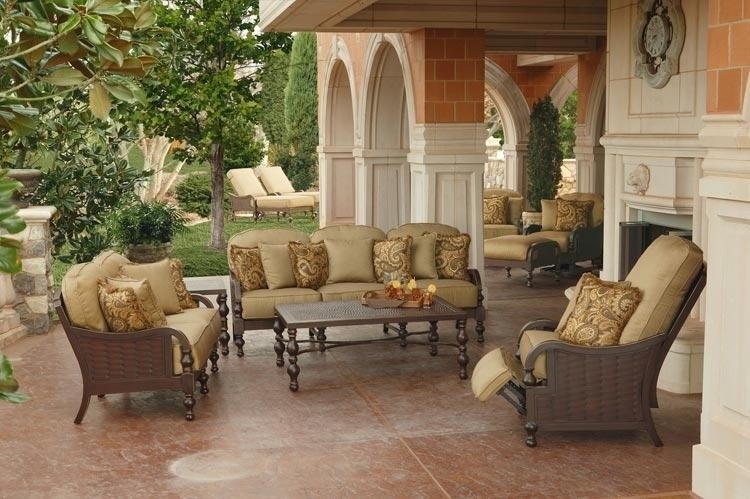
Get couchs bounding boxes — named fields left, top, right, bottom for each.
left=525, top=191, right=607, bottom=277
left=485, top=188, right=528, bottom=238
left=226, top=223, right=487, bottom=358
left=258, top=166, right=320, bottom=216
left=227, top=168, right=316, bottom=225
left=470, top=234, right=709, bottom=447
left=53, top=251, right=229, bottom=425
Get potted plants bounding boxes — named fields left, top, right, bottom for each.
left=522, top=95, right=563, bottom=235
left=106, top=190, right=192, bottom=264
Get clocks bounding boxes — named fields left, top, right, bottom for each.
left=641, top=1, right=672, bottom=73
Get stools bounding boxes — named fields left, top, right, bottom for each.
left=484, top=236, right=562, bottom=287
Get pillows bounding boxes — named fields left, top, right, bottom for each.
left=422, top=233, right=472, bottom=281
left=105, top=274, right=167, bottom=327
left=97, top=279, right=154, bottom=332
left=557, top=275, right=640, bottom=347
left=541, top=199, right=557, bottom=231
left=258, top=239, right=296, bottom=290
left=324, top=237, right=376, bottom=285
left=288, top=239, right=329, bottom=289
left=124, top=258, right=180, bottom=314
left=555, top=197, right=594, bottom=231
left=484, top=193, right=509, bottom=224
left=410, top=233, right=438, bottom=279
left=169, top=258, right=198, bottom=308
left=554, top=272, right=633, bottom=335
left=373, top=235, right=415, bottom=282
left=508, top=196, right=524, bottom=226
left=230, top=243, right=268, bottom=291
